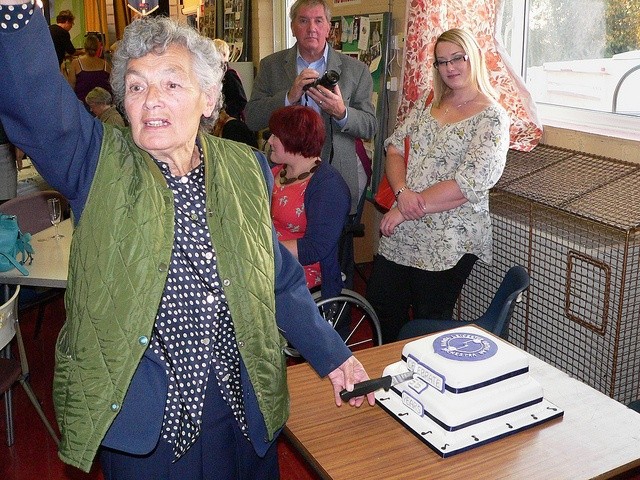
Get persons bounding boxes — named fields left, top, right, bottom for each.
left=243, top=0, right=376, bottom=324
left=262, top=103, right=352, bottom=340
left=66, top=31, right=114, bottom=110
left=85, top=81, right=126, bottom=137
left=42, top=6, right=86, bottom=64
left=0, top=1, right=376, bottom=480
left=205, top=35, right=246, bottom=146
left=374, top=27, right=513, bottom=342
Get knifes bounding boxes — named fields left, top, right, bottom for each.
left=339, top=371, right=414, bottom=402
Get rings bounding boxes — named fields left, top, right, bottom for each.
left=319, top=99, right=323, bottom=104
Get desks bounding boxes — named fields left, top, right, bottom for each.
left=0, top=221, right=72, bottom=288
left=282, top=323, right=640, bottom=480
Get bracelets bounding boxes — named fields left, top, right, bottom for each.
left=394, top=185, right=407, bottom=200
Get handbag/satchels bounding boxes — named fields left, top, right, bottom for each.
left=0, top=214, right=35, bottom=276
left=373, top=89, right=434, bottom=210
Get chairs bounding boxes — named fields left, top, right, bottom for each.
left=1, top=283, right=64, bottom=456
left=1, top=191, right=68, bottom=244
left=398, top=265, right=531, bottom=344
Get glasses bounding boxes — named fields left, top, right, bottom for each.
left=433, top=55, right=469, bottom=70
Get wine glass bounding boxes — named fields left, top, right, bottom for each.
left=47, top=198, right=64, bottom=241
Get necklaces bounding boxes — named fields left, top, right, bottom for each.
left=440, top=91, right=482, bottom=116
left=279, top=157, right=322, bottom=185
